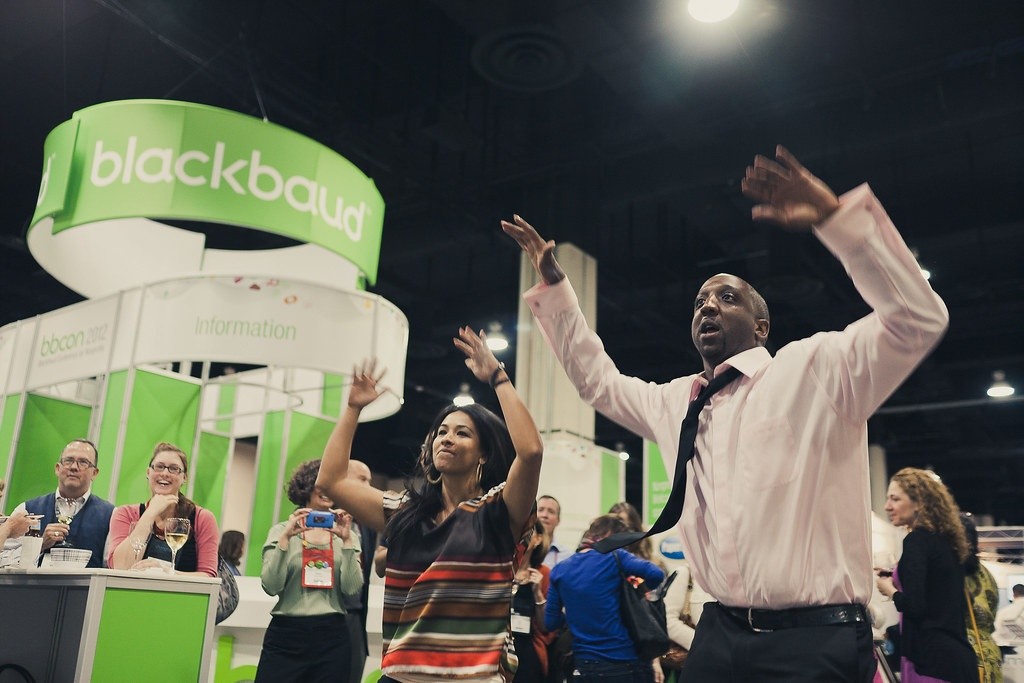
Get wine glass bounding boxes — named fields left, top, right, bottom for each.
left=128, top=521, right=152, bottom=572
left=874, top=548, right=899, bottom=603
left=164, top=517, right=190, bottom=575
left=55, top=497, right=77, bottom=547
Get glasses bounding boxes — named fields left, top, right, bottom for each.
left=150, top=462, right=185, bottom=474
left=60, top=458, right=96, bottom=470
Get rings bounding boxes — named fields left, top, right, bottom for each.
left=55, top=531, right=59, bottom=536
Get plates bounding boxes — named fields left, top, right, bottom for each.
left=0, top=515, right=45, bottom=523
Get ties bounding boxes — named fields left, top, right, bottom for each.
left=593, top=367, right=742, bottom=552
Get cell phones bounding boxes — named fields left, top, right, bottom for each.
left=306, top=512, right=334, bottom=528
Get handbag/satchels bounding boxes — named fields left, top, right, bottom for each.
left=216, top=558, right=238, bottom=624
left=978, top=665, right=991, bottom=683
left=613, top=551, right=670, bottom=661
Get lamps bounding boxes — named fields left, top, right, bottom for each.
left=453, top=382, right=478, bottom=406
left=487, top=321, right=509, bottom=350
left=985, top=370, right=1016, bottom=398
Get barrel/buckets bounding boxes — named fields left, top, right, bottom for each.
left=10, top=537, right=43, bottom=570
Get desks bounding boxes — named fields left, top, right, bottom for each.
left=0, top=567, right=223, bottom=683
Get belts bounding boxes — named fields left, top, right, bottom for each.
left=717, top=600, right=868, bottom=632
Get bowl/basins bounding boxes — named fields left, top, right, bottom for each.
left=51, top=548, right=92, bottom=569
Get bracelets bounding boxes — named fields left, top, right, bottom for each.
left=494, top=379, right=510, bottom=387
left=535, top=600, right=546, bottom=606
left=490, top=362, right=505, bottom=390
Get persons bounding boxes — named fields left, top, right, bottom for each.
left=253, top=458, right=368, bottom=683
left=499, top=144, right=949, bottom=683
left=219, top=530, right=244, bottom=576
left=10, top=439, right=117, bottom=568
left=542, top=502, right=665, bottom=683
left=874, top=464, right=981, bottom=683
left=537, top=495, right=574, bottom=675
left=999, top=584, right=1024, bottom=660
left=315, top=325, right=542, bottom=683
left=960, top=513, right=1003, bottom=683
left=610, top=502, right=669, bottom=683
left=510, top=520, right=560, bottom=683
left=107, top=442, right=219, bottom=577
left=333, top=460, right=377, bottom=683
left=0, top=481, right=38, bottom=552
